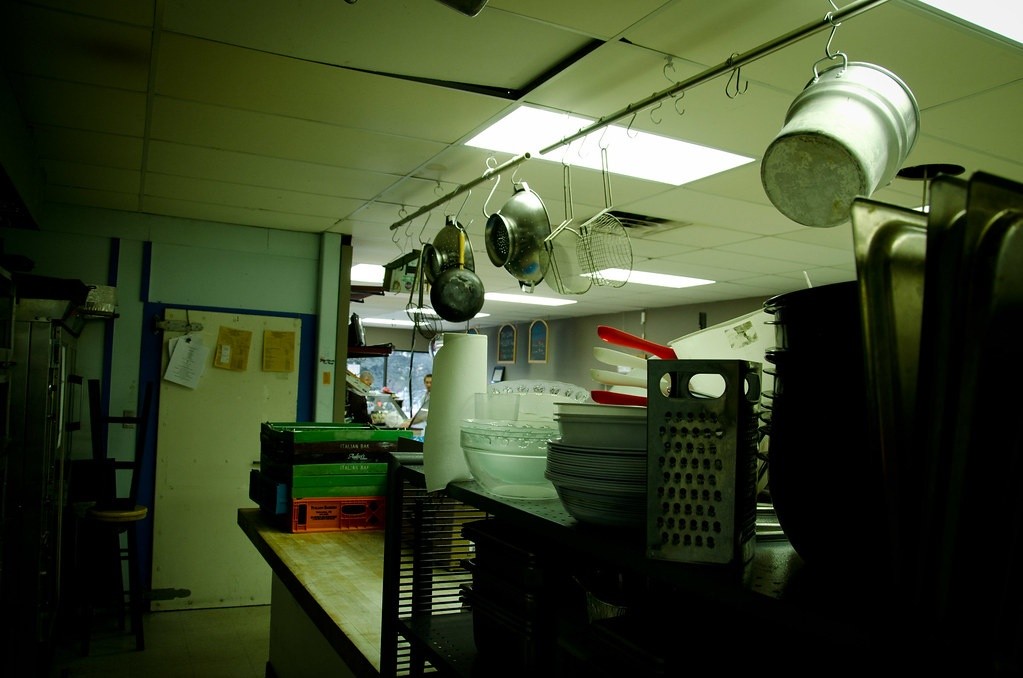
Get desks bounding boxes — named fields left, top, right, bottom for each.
left=236, top=506, right=474, bottom=678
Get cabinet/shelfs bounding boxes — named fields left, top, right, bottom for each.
left=348, top=285, right=394, bottom=356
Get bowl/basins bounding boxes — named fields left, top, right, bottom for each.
left=544, top=401, right=648, bottom=524
left=485, top=380, right=591, bottom=419
left=433, top=214, right=475, bottom=276
left=459, top=419, right=559, bottom=500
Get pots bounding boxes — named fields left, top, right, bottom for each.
left=497, top=178, right=552, bottom=293
left=420, top=239, right=445, bottom=285
left=429, top=230, right=486, bottom=322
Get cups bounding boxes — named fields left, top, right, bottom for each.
left=473, top=392, right=519, bottom=421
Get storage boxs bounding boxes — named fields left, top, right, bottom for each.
left=254, top=422, right=413, bottom=535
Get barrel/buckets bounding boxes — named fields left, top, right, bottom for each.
left=761, top=53, right=917, bottom=228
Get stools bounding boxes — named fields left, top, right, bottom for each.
left=73, top=505, right=147, bottom=655
